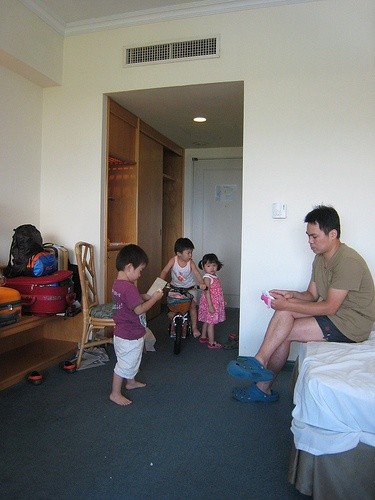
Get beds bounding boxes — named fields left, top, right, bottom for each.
left=288, top=332, right=375, bottom=498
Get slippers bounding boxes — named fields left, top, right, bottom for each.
left=232, top=382, right=279, bottom=402
left=228, top=356, right=275, bottom=382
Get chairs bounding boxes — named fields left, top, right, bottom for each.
left=75, top=242, right=146, bottom=374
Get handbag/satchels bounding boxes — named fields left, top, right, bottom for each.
left=25, top=250, right=57, bottom=277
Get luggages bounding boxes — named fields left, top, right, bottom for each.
left=0, top=287, right=23, bottom=326
left=5, top=270, right=77, bottom=315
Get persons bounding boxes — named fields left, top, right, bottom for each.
left=158, top=237, right=207, bottom=338
left=226, top=204, right=375, bottom=405
left=110, top=244, right=164, bottom=406
left=198, top=253, right=225, bottom=348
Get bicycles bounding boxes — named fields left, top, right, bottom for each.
left=164, top=281, right=208, bottom=355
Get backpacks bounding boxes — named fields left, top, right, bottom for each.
left=10, top=224, right=43, bottom=262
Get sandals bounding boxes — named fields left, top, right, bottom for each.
left=199, top=337, right=209, bottom=343
left=208, top=342, right=222, bottom=348
left=28, top=369, right=42, bottom=385
left=60, top=360, right=76, bottom=374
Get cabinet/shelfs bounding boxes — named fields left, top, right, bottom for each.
left=105, top=100, right=184, bottom=337
left=0, top=316, right=86, bottom=392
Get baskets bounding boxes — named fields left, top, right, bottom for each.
left=167, top=296, right=190, bottom=313
